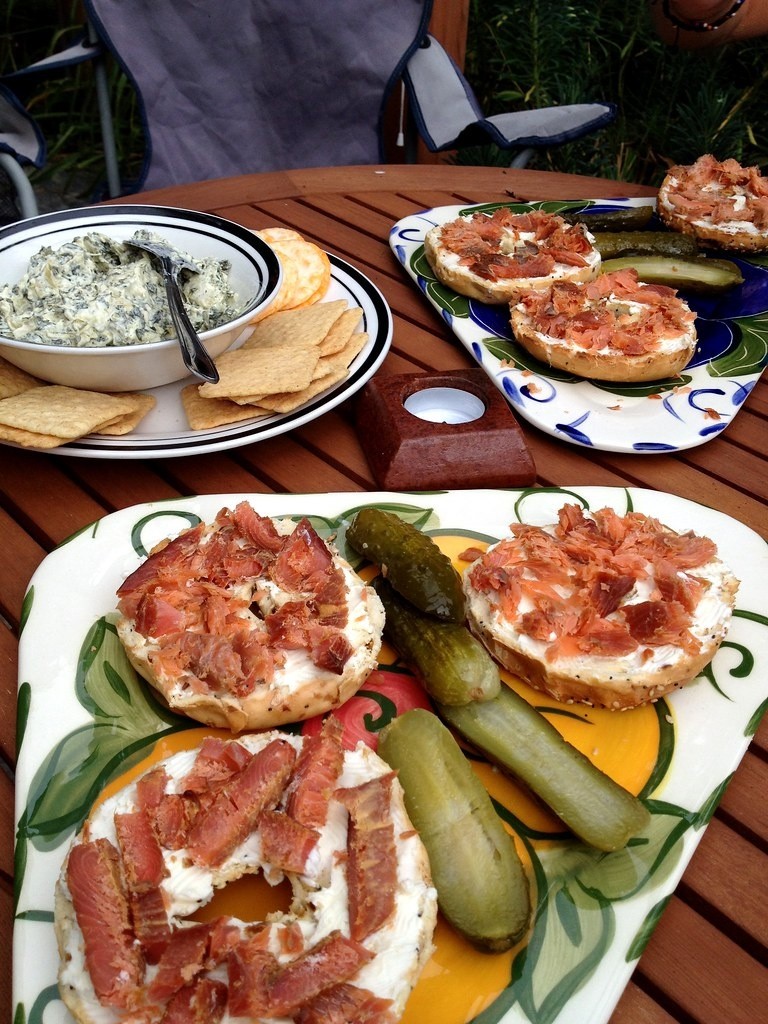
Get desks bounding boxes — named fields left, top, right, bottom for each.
left=0, top=164, right=768, bottom=1024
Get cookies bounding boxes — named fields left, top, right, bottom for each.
left=180, top=300, right=370, bottom=434
left=0, top=357, right=155, bottom=449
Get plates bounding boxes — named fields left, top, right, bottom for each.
left=12, top=484, right=768, bottom=1024
left=0, top=233, right=395, bottom=460
left=390, top=196, right=768, bottom=454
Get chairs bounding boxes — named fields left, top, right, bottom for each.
left=0, top=0, right=615, bottom=220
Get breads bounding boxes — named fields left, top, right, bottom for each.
left=424, top=213, right=602, bottom=302
left=55, top=732, right=434, bottom=1024
left=509, top=280, right=696, bottom=383
left=656, top=173, right=768, bottom=254
left=462, top=545, right=738, bottom=706
left=118, top=546, right=386, bottom=732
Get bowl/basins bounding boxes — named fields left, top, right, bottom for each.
left=0, top=203, right=283, bottom=393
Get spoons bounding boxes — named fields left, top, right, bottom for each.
left=124, top=239, right=220, bottom=384
